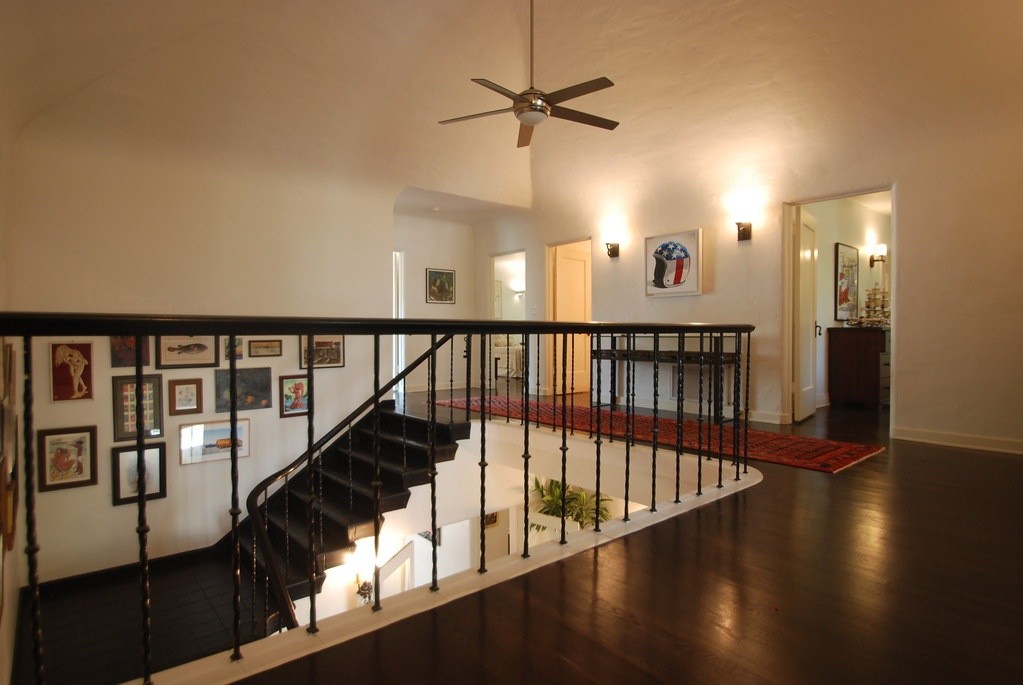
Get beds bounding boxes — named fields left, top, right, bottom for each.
left=492, top=334, right=527, bottom=379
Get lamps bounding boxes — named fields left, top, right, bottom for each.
left=732, top=209, right=752, bottom=242
left=869, top=243, right=888, bottom=267
left=605, top=234, right=620, bottom=258
left=355, top=564, right=373, bottom=606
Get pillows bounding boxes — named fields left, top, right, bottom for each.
left=492, top=334, right=521, bottom=347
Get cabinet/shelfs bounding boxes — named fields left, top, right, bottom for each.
left=827, top=325, right=887, bottom=412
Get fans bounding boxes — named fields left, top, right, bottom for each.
left=438, top=0, right=620, bottom=147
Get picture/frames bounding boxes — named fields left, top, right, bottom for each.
left=835, top=241, right=860, bottom=322
left=38, top=424, right=99, bottom=491
left=111, top=443, right=168, bottom=508
left=178, top=417, right=250, bottom=467
left=299, top=335, right=346, bottom=370
left=49, top=340, right=96, bottom=403
left=248, top=340, right=283, bottom=357
left=426, top=267, right=456, bottom=305
left=483, top=512, right=500, bottom=529
left=417, top=526, right=441, bottom=547
left=169, top=378, right=204, bottom=416
left=112, top=372, right=166, bottom=442
left=156, top=333, right=220, bottom=368
left=279, top=374, right=312, bottom=419
left=644, top=227, right=703, bottom=298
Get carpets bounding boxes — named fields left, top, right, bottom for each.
left=431, top=391, right=888, bottom=475
